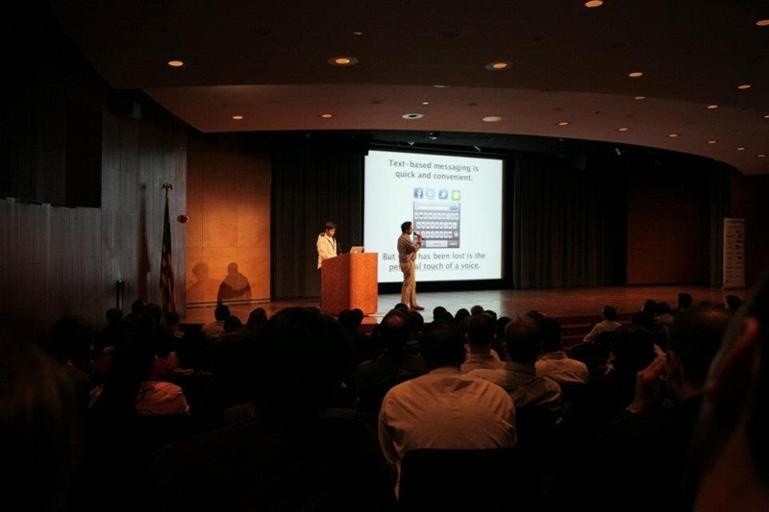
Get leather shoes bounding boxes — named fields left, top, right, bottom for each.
left=411, top=306, right=424, bottom=310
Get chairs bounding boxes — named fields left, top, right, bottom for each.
left=398, top=448, right=518, bottom=510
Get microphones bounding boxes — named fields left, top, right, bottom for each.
left=413, top=230, right=426, bottom=242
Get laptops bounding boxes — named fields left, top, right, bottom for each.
left=349, top=246, right=364, bottom=253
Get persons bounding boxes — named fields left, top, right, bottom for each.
left=314, top=222, right=340, bottom=271
left=1, top=282, right=768, bottom=510
left=396, top=221, right=425, bottom=312
